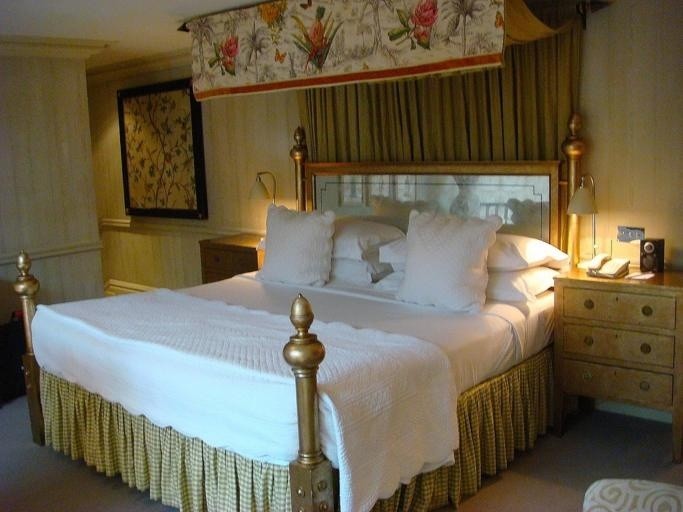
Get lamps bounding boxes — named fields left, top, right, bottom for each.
left=566, top=173, right=598, bottom=269
left=248, top=171, right=276, bottom=241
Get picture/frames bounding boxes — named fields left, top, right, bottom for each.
left=116, top=77, right=208, bottom=220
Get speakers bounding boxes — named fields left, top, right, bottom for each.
left=639, top=238, right=664, bottom=273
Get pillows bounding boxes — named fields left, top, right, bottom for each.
left=373, top=267, right=561, bottom=304
left=378, top=228, right=569, bottom=272
left=393, top=208, right=505, bottom=312
left=324, top=255, right=388, bottom=286
left=264, top=204, right=334, bottom=290
left=325, top=218, right=405, bottom=261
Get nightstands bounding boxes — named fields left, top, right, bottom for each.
left=199, top=232, right=264, bottom=284
left=553, top=267, right=683, bottom=464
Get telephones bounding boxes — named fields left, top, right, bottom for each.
left=587, top=254, right=630, bottom=278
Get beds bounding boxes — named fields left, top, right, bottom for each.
left=13, top=112, right=587, bottom=512
